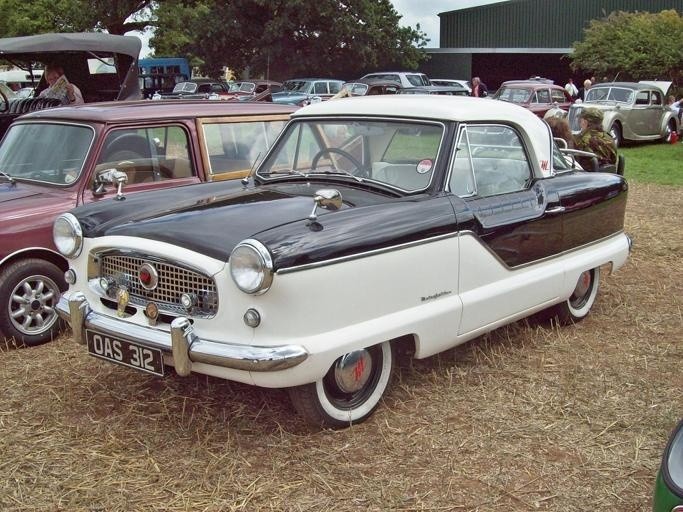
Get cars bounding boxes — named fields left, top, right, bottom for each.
left=52, top=94, right=633, bottom=431
left=544, top=80, right=680, bottom=147
left=489, top=75, right=575, bottom=118
left=0, top=99, right=341, bottom=350
left=139, top=70, right=473, bottom=105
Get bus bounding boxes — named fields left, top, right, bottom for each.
left=137, top=55, right=192, bottom=83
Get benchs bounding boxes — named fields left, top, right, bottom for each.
left=0, top=99, right=60, bottom=115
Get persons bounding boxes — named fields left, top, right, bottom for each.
left=470, top=76, right=490, bottom=97
left=572, top=105, right=620, bottom=173
left=574, top=79, right=592, bottom=101
left=543, top=107, right=578, bottom=158
left=8, top=83, right=20, bottom=91
left=37, top=61, right=85, bottom=106
left=564, top=78, right=579, bottom=98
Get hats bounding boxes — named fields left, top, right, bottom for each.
left=574, top=107, right=604, bottom=121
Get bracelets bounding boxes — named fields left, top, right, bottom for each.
left=70, top=95, right=76, bottom=102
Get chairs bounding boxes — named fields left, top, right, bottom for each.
left=87, top=156, right=190, bottom=184
left=376, top=163, right=508, bottom=196
